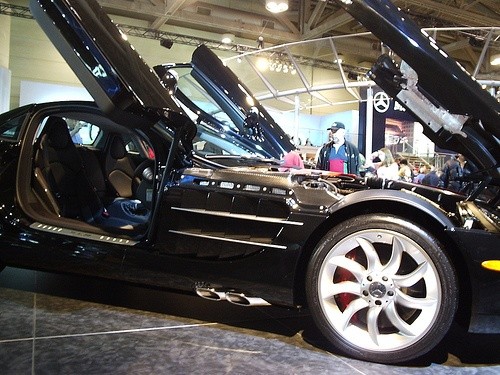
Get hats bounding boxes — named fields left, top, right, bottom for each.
left=327, top=122, right=345, bottom=130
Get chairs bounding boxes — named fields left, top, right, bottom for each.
left=32, top=115, right=138, bottom=224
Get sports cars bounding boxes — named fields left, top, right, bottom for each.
left=0, top=0, right=500, bottom=367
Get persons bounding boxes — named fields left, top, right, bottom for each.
left=357, top=152, right=369, bottom=177
left=66, top=118, right=83, bottom=145
left=290, top=136, right=313, bottom=146
left=412, top=154, right=465, bottom=188
left=365, top=148, right=399, bottom=189
left=316, top=122, right=360, bottom=176
left=398, top=159, right=412, bottom=182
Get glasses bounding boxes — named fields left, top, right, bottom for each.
left=331, top=129, right=338, bottom=133
